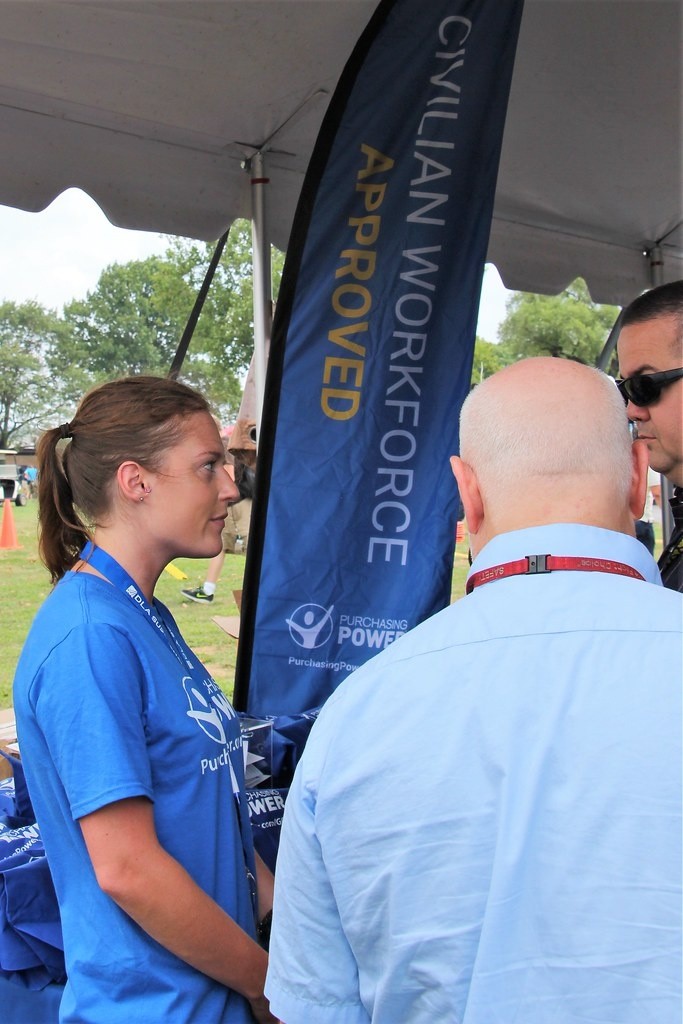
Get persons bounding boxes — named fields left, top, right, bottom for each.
left=11, top=376, right=277, bottom=1024
left=262, top=355, right=682, bottom=1024
left=616, top=280, right=682, bottom=593
left=180, top=453, right=258, bottom=603
left=24, top=466, right=38, bottom=488
left=631, top=427, right=663, bottom=556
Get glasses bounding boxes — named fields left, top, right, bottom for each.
left=616, top=364, right=683, bottom=408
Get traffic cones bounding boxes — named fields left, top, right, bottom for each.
left=0, top=499, right=25, bottom=549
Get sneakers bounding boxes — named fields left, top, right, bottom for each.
left=181, top=585, right=215, bottom=604
